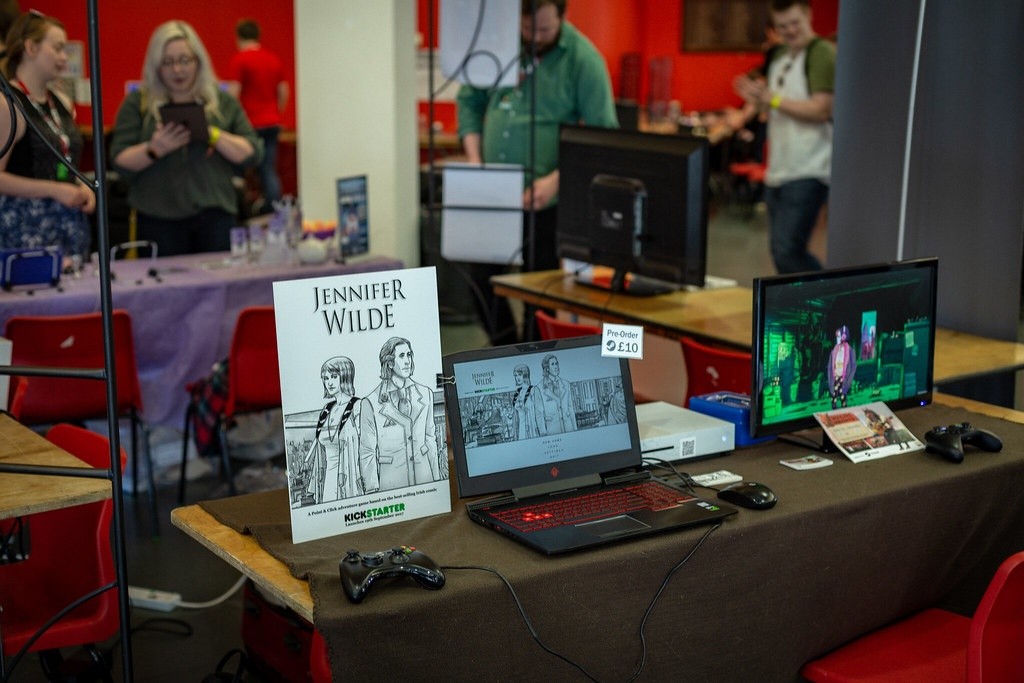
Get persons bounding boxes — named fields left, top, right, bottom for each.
left=1, top=11, right=99, bottom=269
left=457, top=0, right=620, bottom=344
left=228, top=20, right=288, bottom=212
left=110, top=20, right=263, bottom=258
left=864, top=409, right=900, bottom=445
left=711, top=0, right=835, bottom=275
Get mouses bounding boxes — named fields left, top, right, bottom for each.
left=717, top=482, right=777, bottom=512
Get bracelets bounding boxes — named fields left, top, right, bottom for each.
left=207, top=126, right=219, bottom=144
left=147, top=141, right=160, bottom=162
left=770, top=95, right=782, bottom=108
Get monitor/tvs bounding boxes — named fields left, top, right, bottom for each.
left=752, top=258, right=939, bottom=453
left=556, top=120, right=712, bottom=296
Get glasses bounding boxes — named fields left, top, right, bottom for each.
left=162, top=54, right=197, bottom=67
left=777, top=62, right=792, bottom=86
left=20, top=8, right=44, bottom=41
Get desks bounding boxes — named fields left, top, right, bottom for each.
left=491, top=256, right=1023, bottom=426
left=0, top=245, right=415, bottom=495
left=169, top=393, right=1023, bottom=683
left=1, top=413, right=114, bottom=525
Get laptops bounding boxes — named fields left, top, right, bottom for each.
left=441, top=334, right=739, bottom=557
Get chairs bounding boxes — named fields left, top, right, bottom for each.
left=6, top=308, right=161, bottom=539
left=800, top=552, right=1024, bottom=683
left=175, top=305, right=278, bottom=510
left=534, top=310, right=602, bottom=340
left=0, top=422, right=127, bottom=681
left=680, top=338, right=753, bottom=409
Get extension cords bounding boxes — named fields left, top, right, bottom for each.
left=128, top=586, right=180, bottom=612
